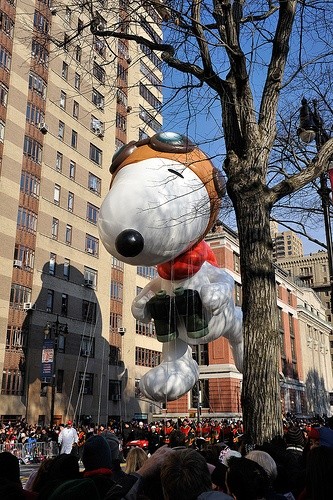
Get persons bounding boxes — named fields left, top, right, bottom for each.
left=0, top=412, right=333, bottom=500
left=57, top=420, right=79, bottom=455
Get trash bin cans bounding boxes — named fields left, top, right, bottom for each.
left=125, top=440, right=149, bottom=454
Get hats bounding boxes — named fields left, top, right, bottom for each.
left=139, top=422, right=143, bottom=426
left=68, top=420, right=72, bottom=425
left=101, top=432, right=119, bottom=459
left=308, top=427, right=333, bottom=447
left=82, top=435, right=110, bottom=468
left=151, top=426, right=156, bottom=429
left=285, top=427, right=304, bottom=445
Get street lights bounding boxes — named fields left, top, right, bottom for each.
left=45, top=312, right=70, bottom=431
left=295, top=97, right=333, bottom=316
